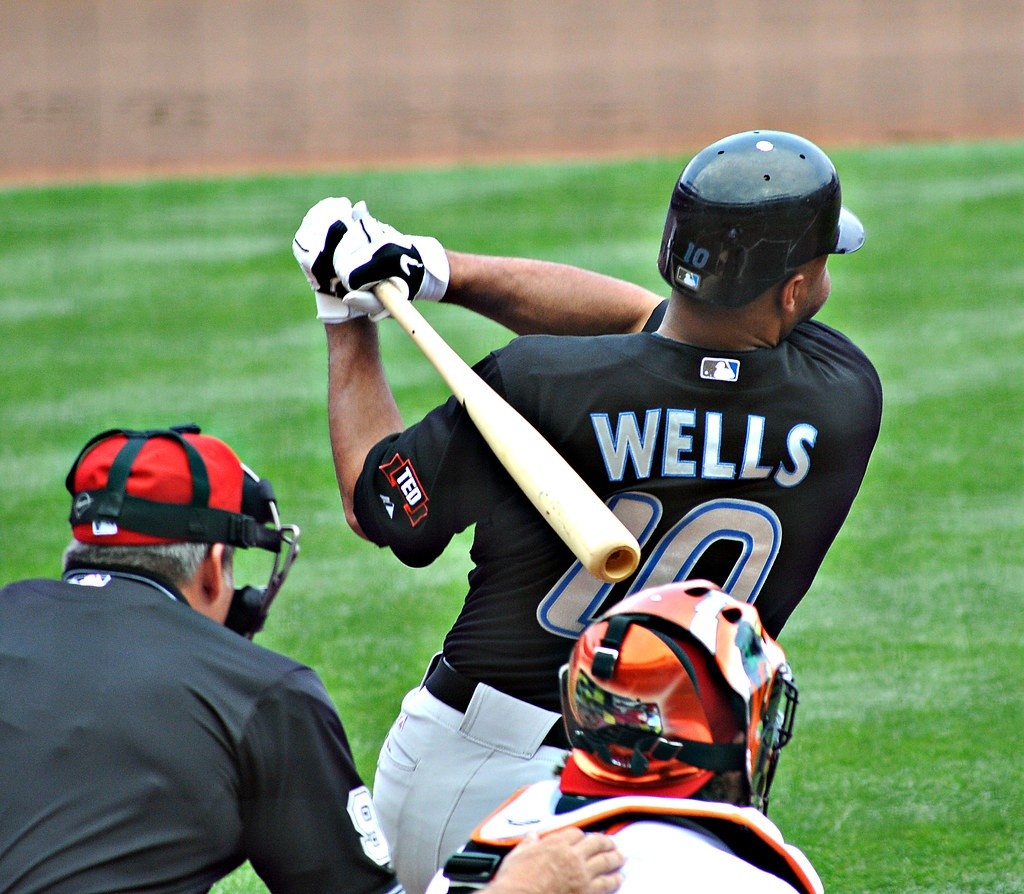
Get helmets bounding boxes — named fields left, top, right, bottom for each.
left=656, top=128, right=868, bottom=311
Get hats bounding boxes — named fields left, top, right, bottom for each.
left=62, top=426, right=249, bottom=548
left=558, top=621, right=737, bottom=795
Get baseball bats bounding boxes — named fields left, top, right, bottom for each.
left=371, top=280, right=641, bottom=582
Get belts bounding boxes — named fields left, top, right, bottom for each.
left=424, top=656, right=571, bottom=759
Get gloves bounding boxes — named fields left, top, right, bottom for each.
left=331, top=198, right=451, bottom=315
left=292, top=195, right=378, bottom=326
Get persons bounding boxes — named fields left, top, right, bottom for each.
left=292, top=131, right=882, bottom=894
left=0, top=425, right=408, bottom=894
